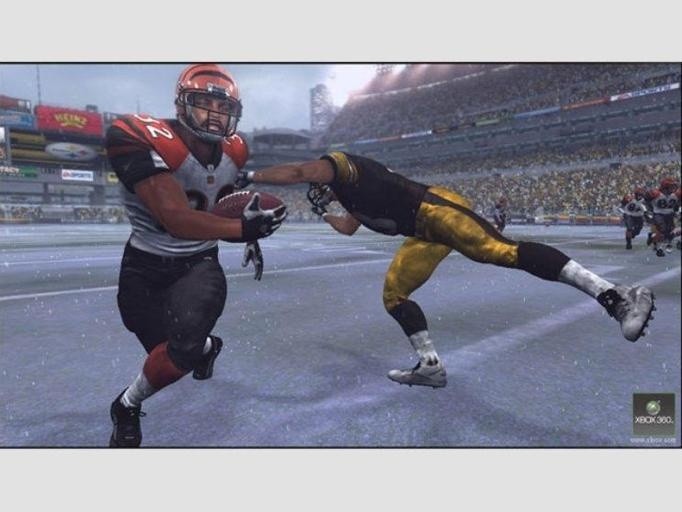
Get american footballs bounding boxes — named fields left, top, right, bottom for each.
left=212, top=192, right=285, bottom=242
